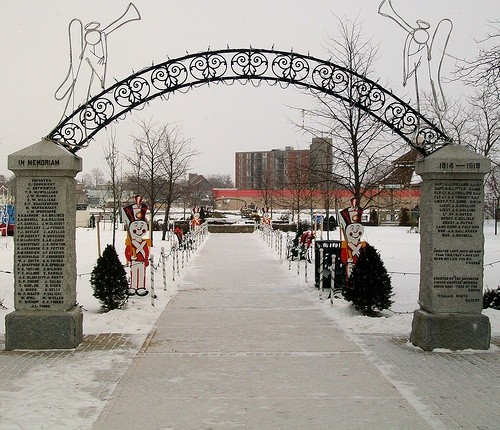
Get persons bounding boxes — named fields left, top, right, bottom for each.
left=190, top=207, right=202, bottom=227
left=123, top=195, right=152, bottom=297
left=339, top=198, right=368, bottom=287
left=262, top=205, right=271, bottom=225
left=90, top=213, right=97, bottom=228
